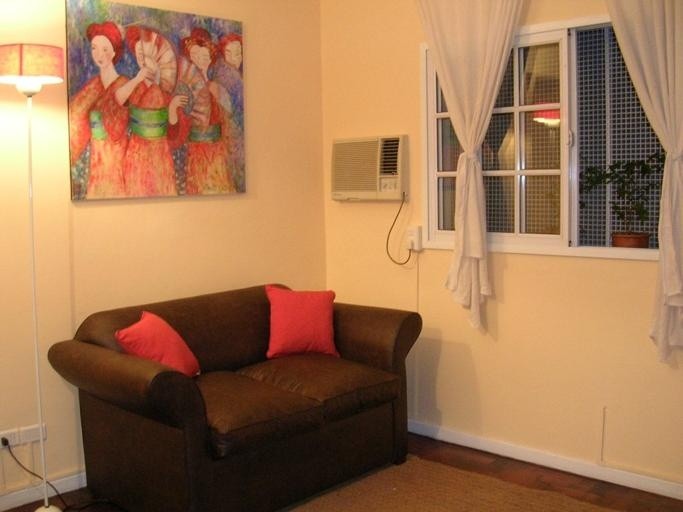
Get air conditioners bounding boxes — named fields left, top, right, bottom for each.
left=331, top=134, right=409, bottom=201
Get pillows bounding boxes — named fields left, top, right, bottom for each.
left=114, top=310, right=202, bottom=380
left=264, top=284, right=341, bottom=360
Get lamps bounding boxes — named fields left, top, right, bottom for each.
left=0, top=42, right=65, bottom=512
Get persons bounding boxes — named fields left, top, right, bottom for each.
left=168, top=25, right=241, bottom=195
left=115, top=23, right=192, bottom=197
left=67, top=21, right=132, bottom=201
left=211, top=31, right=246, bottom=194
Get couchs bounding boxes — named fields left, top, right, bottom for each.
left=49, top=285, right=424, bottom=512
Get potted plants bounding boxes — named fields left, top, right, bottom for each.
left=576, top=151, right=665, bottom=247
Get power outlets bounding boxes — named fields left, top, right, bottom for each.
left=1, top=429, right=19, bottom=448
left=405, top=225, right=422, bottom=251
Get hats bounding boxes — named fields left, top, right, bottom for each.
left=219, top=33, right=243, bottom=57
left=86, top=19, right=123, bottom=65
left=126, top=24, right=143, bottom=56
left=179, top=27, right=219, bottom=66
